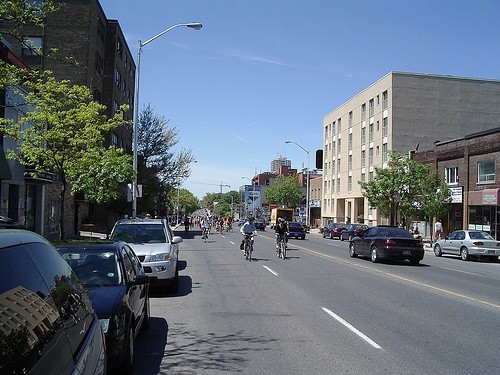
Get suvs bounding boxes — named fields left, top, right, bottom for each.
left=0, top=215, right=108, bottom=375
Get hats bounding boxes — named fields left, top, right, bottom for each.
left=279, top=218, right=286, bottom=224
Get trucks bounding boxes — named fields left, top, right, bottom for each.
left=270, top=208, right=295, bottom=230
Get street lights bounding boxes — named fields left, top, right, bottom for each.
left=176, top=160, right=197, bottom=223
left=285, top=140, right=311, bottom=234
left=241, top=177, right=255, bottom=217
left=131, top=21, right=204, bottom=219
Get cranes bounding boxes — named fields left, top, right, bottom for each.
left=190, top=179, right=231, bottom=194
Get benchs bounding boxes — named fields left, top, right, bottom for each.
left=69, top=255, right=114, bottom=274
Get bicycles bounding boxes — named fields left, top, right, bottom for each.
left=203, top=227, right=209, bottom=243
left=242, top=234, right=258, bottom=262
left=274, top=232, right=288, bottom=260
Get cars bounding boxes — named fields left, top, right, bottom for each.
left=339, top=223, right=370, bottom=242
left=108, top=213, right=184, bottom=296
left=321, top=223, right=347, bottom=239
left=347, top=224, right=425, bottom=266
left=287, top=221, right=306, bottom=240
left=433, top=229, right=500, bottom=261
left=55, top=239, right=151, bottom=375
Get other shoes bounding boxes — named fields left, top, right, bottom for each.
left=244, top=251, right=246, bottom=255
left=250, top=247, right=253, bottom=251
left=286, top=243, right=288, bottom=247
left=276, top=244, right=279, bottom=248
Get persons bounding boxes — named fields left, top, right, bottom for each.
left=146, top=214, right=150, bottom=219
left=183, top=215, right=233, bottom=239
left=61, top=293, right=86, bottom=317
left=397, top=220, right=403, bottom=226
left=240, top=218, right=257, bottom=256
left=409, top=226, right=421, bottom=239
left=275, top=218, right=288, bottom=252
left=435, top=219, right=443, bottom=238
